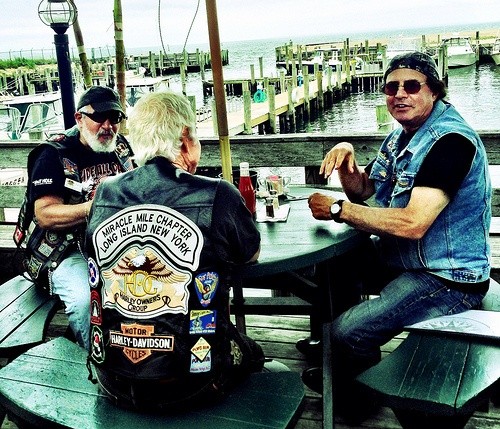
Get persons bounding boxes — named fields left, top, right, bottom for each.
left=296, top=54, right=492, bottom=403
left=13, top=87, right=136, bottom=349
left=86, top=90, right=264, bottom=413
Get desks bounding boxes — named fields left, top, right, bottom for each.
left=231, top=188, right=370, bottom=429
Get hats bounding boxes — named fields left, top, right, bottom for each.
left=77, top=86, right=128, bottom=120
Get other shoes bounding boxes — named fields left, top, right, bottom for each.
left=302, top=366, right=324, bottom=395
left=295, top=338, right=353, bottom=368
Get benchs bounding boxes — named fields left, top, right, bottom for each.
left=0, top=271, right=499, bottom=429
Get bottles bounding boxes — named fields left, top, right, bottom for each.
left=265, top=198, right=274, bottom=218
left=269, top=190, right=280, bottom=210
left=239, top=161, right=256, bottom=213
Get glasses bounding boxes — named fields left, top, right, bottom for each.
left=81, top=110, right=124, bottom=125
left=379, top=78, right=427, bottom=96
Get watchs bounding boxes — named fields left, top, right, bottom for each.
left=330, top=200, right=346, bottom=224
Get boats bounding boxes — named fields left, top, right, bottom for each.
left=275, top=50, right=352, bottom=72
left=489, top=38, right=500, bottom=65
left=431, top=36, right=475, bottom=66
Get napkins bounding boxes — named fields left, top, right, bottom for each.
left=255, top=203, right=290, bottom=223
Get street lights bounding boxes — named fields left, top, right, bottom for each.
left=37, top=0, right=77, bottom=130
